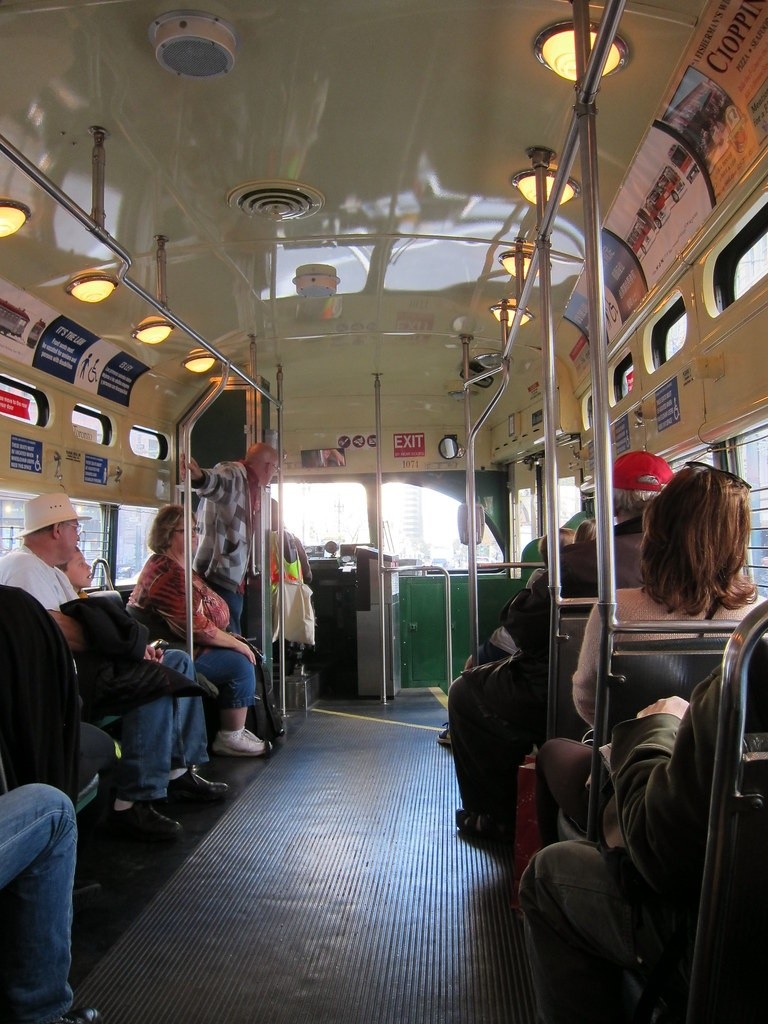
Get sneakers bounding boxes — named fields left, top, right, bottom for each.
left=212, top=730, right=272, bottom=757
left=52, top=1005, right=107, bottom=1024
left=436, top=728, right=451, bottom=743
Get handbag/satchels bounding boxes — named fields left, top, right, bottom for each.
left=271, top=579, right=315, bottom=646
left=537, top=738, right=632, bottom=854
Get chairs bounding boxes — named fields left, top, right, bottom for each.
left=540, top=592, right=768, bottom=1024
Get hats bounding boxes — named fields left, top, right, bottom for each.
left=613, top=452, right=675, bottom=491
left=12, top=492, right=93, bottom=540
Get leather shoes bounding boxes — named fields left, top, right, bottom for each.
left=170, top=769, right=230, bottom=797
left=101, top=802, right=178, bottom=840
left=456, top=808, right=507, bottom=841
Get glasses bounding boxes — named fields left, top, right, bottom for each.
left=174, top=527, right=203, bottom=534
left=58, top=520, right=83, bottom=535
left=673, top=460, right=751, bottom=501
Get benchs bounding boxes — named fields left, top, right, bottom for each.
left=1, top=581, right=137, bottom=815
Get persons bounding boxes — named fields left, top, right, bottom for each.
left=0, top=492, right=226, bottom=837
left=268, top=497, right=313, bottom=585
left=0, top=784, right=102, bottom=1024
left=518, top=462, right=767, bottom=848
left=434, top=528, right=578, bottom=747
left=575, top=521, right=597, bottom=550
left=123, top=505, right=274, bottom=755
left=518, top=642, right=768, bottom=1024
left=446, top=450, right=677, bottom=845
left=176, top=444, right=281, bottom=637
left=57, top=544, right=95, bottom=596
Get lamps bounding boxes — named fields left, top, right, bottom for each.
left=533, top=20, right=630, bottom=82
left=65, top=269, right=120, bottom=305
left=512, top=163, right=581, bottom=209
left=131, top=316, right=175, bottom=344
left=0, top=197, right=30, bottom=238
left=182, top=348, right=217, bottom=373
left=489, top=298, right=535, bottom=327
left=495, top=243, right=552, bottom=284
